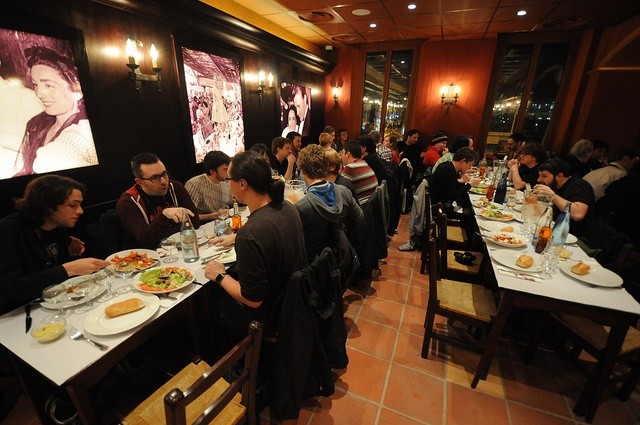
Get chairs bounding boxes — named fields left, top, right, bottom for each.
left=117, top=321, right=262, bottom=425
left=424, top=179, right=460, bottom=224
left=420, top=187, right=469, bottom=274
left=522, top=244, right=640, bottom=416
left=99, top=209, right=123, bottom=255
left=420, top=223, right=497, bottom=381
left=231, top=331, right=284, bottom=424
left=436, top=202, right=483, bottom=324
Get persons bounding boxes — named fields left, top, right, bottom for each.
left=506, top=143, right=543, bottom=191
left=594, top=160, right=640, bottom=246
left=115, top=152, right=200, bottom=249
left=323, top=125, right=338, bottom=148
left=323, top=148, right=359, bottom=208
left=586, top=141, right=609, bottom=171
left=582, top=148, right=640, bottom=202
left=561, top=138, right=594, bottom=178
left=432, top=135, right=474, bottom=184
left=483, top=133, right=539, bottom=169
left=420, top=130, right=447, bottom=158
left=281, top=106, right=300, bottom=137
left=0, top=175, right=112, bottom=313
left=367, top=130, right=393, bottom=177
left=530, top=157, right=595, bottom=256
left=248, top=143, right=279, bottom=180
left=503, top=142, right=510, bottom=161
left=383, top=134, right=400, bottom=166
left=204, top=151, right=308, bottom=339
left=13, top=45, right=89, bottom=180
left=269, top=137, right=300, bottom=184
left=294, top=144, right=364, bottom=282
left=356, top=137, right=387, bottom=186
left=337, top=128, right=348, bottom=146
left=184, top=151, right=241, bottom=220
left=423, top=132, right=449, bottom=168
left=186, top=91, right=243, bottom=141
left=286, top=132, right=302, bottom=151
left=318, top=132, right=333, bottom=148
left=397, top=129, right=427, bottom=185
left=293, top=87, right=310, bottom=136
left=429, top=146, right=482, bottom=236
left=334, top=140, right=379, bottom=210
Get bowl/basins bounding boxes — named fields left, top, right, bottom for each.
left=30, top=316, right=67, bottom=343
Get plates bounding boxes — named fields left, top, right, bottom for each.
left=470, top=182, right=491, bottom=189
left=485, top=232, right=530, bottom=248
left=131, top=265, right=196, bottom=295
left=228, top=207, right=251, bottom=217
left=82, top=291, right=160, bottom=336
left=105, top=248, right=160, bottom=272
left=559, top=261, right=625, bottom=288
left=513, top=212, right=556, bottom=225
left=562, top=232, right=578, bottom=244
left=472, top=200, right=505, bottom=210
left=160, top=230, right=213, bottom=249
left=479, top=208, right=515, bottom=222
left=467, top=187, right=495, bottom=195
left=479, top=220, right=520, bottom=233
left=513, top=205, right=530, bottom=212
left=39, top=273, right=108, bottom=310
left=200, top=244, right=236, bottom=264
left=490, top=248, right=545, bottom=272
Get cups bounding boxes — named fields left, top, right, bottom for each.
left=283, top=179, right=307, bottom=205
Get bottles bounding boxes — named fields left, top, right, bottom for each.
left=231, top=203, right=242, bottom=234
left=223, top=220, right=232, bottom=247
left=479, top=153, right=487, bottom=179
left=486, top=178, right=495, bottom=202
left=494, top=171, right=508, bottom=205
left=531, top=199, right=554, bottom=247
left=550, top=201, right=573, bottom=258
left=180, top=208, right=199, bottom=263
left=535, top=215, right=553, bottom=254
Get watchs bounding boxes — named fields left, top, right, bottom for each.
left=215, top=272, right=228, bottom=286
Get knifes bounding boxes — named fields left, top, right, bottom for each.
left=499, top=268, right=545, bottom=282
left=24, top=304, right=33, bottom=333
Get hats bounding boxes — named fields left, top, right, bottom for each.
left=430, top=133, right=448, bottom=145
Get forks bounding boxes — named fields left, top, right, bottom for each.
left=63, top=324, right=109, bottom=352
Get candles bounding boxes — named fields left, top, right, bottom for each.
left=456, top=86, right=458, bottom=96
left=127, top=39, right=137, bottom=65
left=268, top=72, right=273, bottom=86
left=150, top=44, right=159, bottom=67
left=259, top=72, right=265, bottom=85
left=334, top=90, right=337, bottom=97
left=442, top=87, right=445, bottom=97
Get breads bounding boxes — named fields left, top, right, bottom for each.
left=570, top=262, right=589, bottom=278
left=515, top=254, right=533, bottom=271
left=502, top=226, right=515, bottom=233
left=105, top=298, right=147, bottom=316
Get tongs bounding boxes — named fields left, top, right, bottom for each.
left=200, top=249, right=232, bottom=265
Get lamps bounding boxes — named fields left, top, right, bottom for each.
left=332, top=86, right=339, bottom=109
left=125, top=39, right=162, bottom=94
left=440, top=83, right=459, bottom=112
left=254, top=71, right=275, bottom=102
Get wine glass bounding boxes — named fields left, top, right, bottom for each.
left=505, top=197, right=516, bottom=212
left=42, top=283, right=72, bottom=319
left=518, top=218, right=535, bottom=238
left=203, top=226, right=217, bottom=249
left=160, top=237, right=178, bottom=263
left=114, top=261, right=135, bottom=293
left=217, top=208, right=228, bottom=222
left=95, top=264, right=119, bottom=301
left=73, top=276, right=99, bottom=313
left=541, top=239, right=563, bottom=280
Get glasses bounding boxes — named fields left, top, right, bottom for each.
left=224, top=177, right=244, bottom=183
left=521, top=151, right=536, bottom=157
left=134, top=170, right=171, bottom=184
